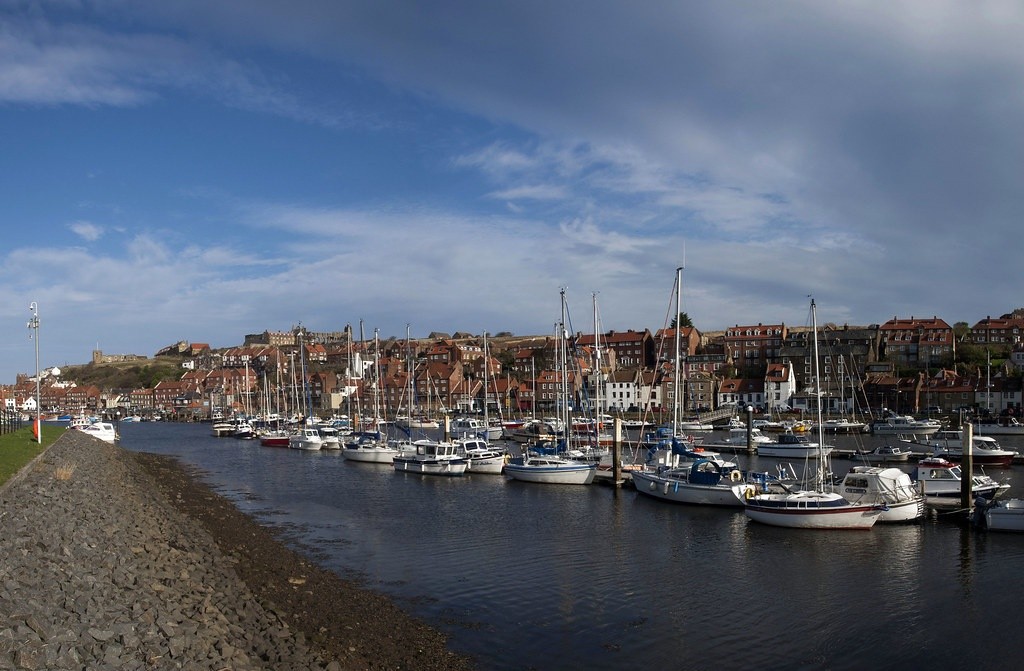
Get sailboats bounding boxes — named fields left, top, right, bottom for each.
left=210, top=265, right=1024, bottom=532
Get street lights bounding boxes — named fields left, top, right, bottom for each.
left=27, top=302, right=41, bottom=444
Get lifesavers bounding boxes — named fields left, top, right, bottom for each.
left=745, top=488, right=755, bottom=499
left=693, top=448, right=704, bottom=452
left=730, top=469, right=741, bottom=482
left=504, top=455, right=511, bottom=465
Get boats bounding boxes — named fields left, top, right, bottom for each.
left=121, top=413, right=164, bottom=423
left=68, top=409, right=122, bottom=446
